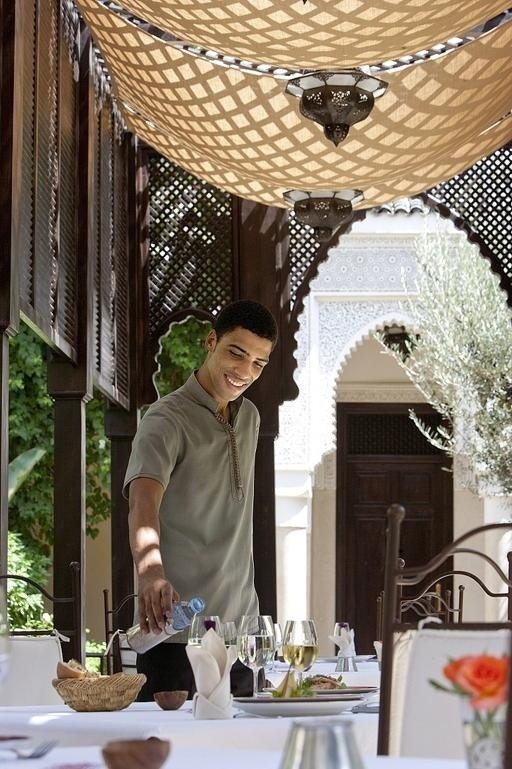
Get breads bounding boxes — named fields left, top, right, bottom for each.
left=57, top=660, right=85, bottom=678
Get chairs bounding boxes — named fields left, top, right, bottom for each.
left=375, top=501, right=512, bottom=759
left=1, top=558, right=142, bottom=707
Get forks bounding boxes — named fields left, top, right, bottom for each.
left=0, top=738, right=58, bottom=759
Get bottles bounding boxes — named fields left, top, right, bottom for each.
left=125, top=598, right=206, bottom=655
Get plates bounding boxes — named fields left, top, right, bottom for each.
left=324, top=655, right=376, bottom=663
left=232, top=699, right=364, bottom=717
left=311, top=685, right=378, bottom=695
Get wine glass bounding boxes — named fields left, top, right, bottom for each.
left=281, top=619, right=319, bottom=689
left=236, top=615, right=277, bottom=696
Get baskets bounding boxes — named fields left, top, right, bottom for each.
left=52, top=673, right=147, bottom=711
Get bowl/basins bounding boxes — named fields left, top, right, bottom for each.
left=153, top=690, right=189, bottom=710
left=101, top=739, right=170, bottom=769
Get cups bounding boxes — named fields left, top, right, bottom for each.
left=187, top=614, right=221, bottom=647
left=334, top=622, right=350, bottom=637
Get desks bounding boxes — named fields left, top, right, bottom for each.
left=2, top=651, right=466, bottom=768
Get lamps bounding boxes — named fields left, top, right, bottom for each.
left=286, top=69, right=391, bottom=153
left=282, top=189, right=365, bottom=251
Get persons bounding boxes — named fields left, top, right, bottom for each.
left=121, top=300, right=279, bottom=660
